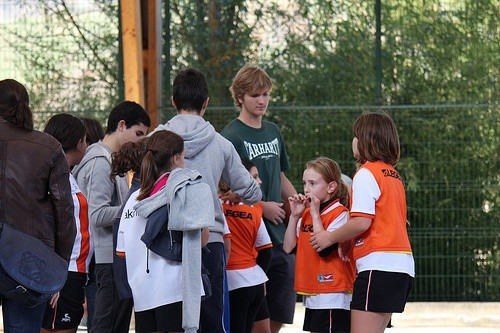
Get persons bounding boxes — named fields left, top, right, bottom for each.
left=43, top=65, right=358, bottom=333
left=309, top=112, right=415, bottom=333
left=0, top=79, right=77, bottom=333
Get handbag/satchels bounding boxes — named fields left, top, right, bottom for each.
left=0, top=222, right=69, bottom=310
